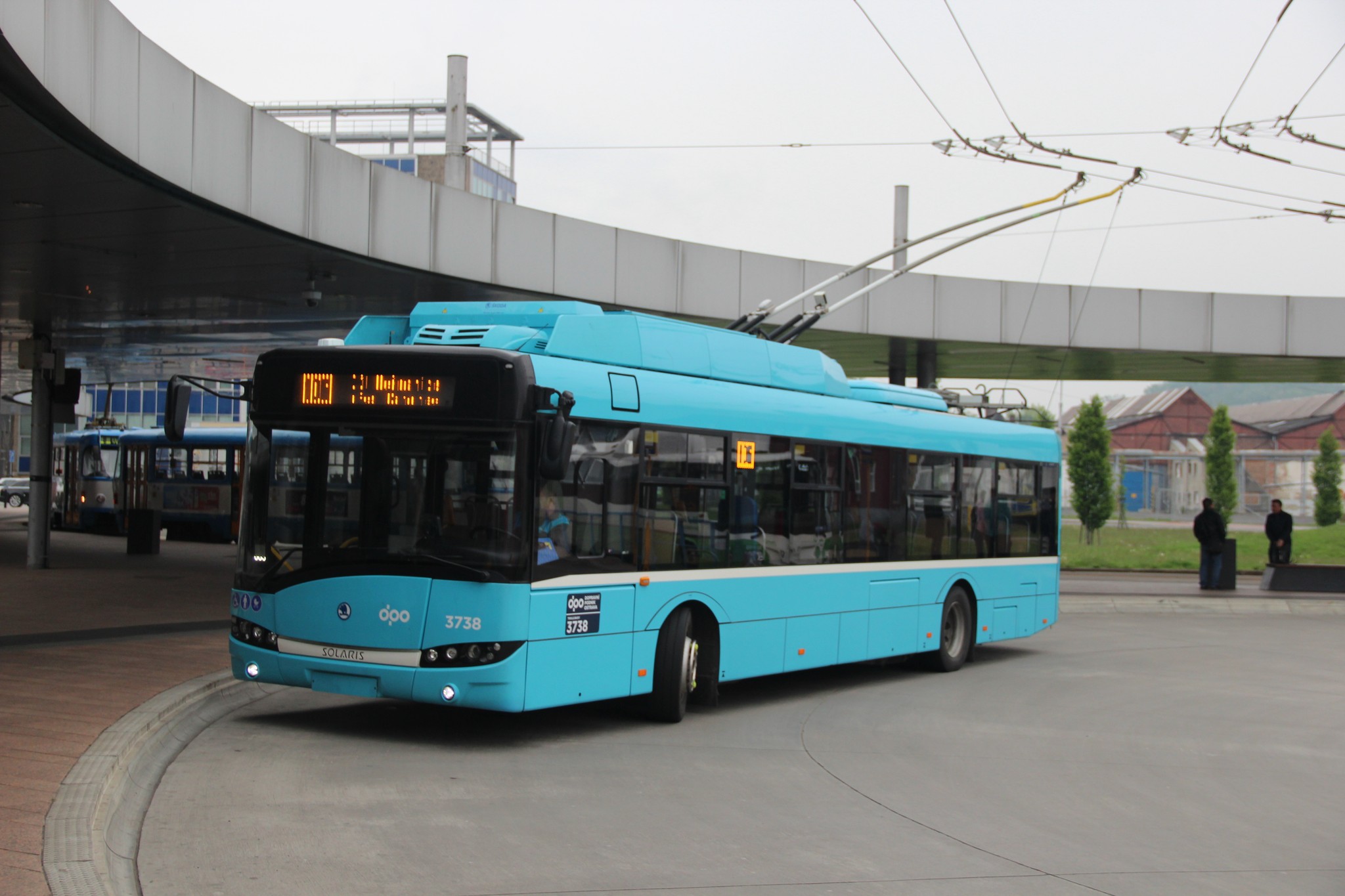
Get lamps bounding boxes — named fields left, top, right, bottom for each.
left=85, top=383, right=124, bottom=432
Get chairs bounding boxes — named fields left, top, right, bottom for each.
left=550, top=514, right=1031, bottom=565
left=157, top=468, right=224, bottom=479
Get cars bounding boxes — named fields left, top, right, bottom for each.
left=0, top=477, right=30, bottom=507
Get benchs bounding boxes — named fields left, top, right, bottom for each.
left=1260, top=564, right=1345, bottom=592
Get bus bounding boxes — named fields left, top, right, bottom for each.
left=48, top=417, right=364, bottom=547
left=221, top=164, right=1145, bottom=726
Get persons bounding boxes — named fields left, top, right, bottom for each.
left=538, top=488, right=572, bottom=567
left=1193, top=497, right=1227, bottom=589
left=1265, top=499, right=1293, bottom=564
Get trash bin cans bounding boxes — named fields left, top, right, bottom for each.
left=123, top=509, right=160, bottom=553
left=1202, top=538, right=1237, bottom=591
